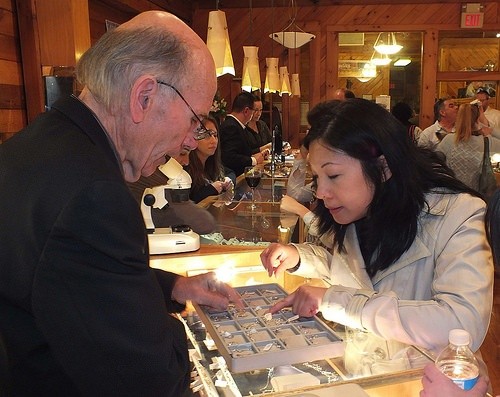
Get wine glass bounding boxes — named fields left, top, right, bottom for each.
left=245, top=167, right=261, bottom=209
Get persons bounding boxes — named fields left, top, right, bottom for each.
left=475, top=91, right=500, bottom=138
left=183, top=113, right=237, bottom=202
left=259, top=97, right=494, bottom=395
left=0, top=8, right=245, bottom=396
left=434, top=100, right=499, bottom=194
left=218, top=90, right=269, bottom=183
left=283, top=140, right=317, bottom=201
left=334, top=88, right=355, bottom=99
left=390, top=102, right=425, bottom=144
left=122, top=140, right=213, bottom=231
left=244, top=94, right=291, bottom=159
left=417, top=98, right=460, bottom=153
left=419, top=364, right=491, bottom=396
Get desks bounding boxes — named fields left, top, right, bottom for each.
left=145, top=144, right=494, bottom=397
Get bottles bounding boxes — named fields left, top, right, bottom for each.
left=435, top=329, right=480, bottom=391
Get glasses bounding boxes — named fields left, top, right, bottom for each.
left=156, top=80, right=215, bottom=141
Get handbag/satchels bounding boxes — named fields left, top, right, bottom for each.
left=479, top=137, right=497, bottom=201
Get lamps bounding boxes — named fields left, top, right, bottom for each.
left=268, top=0, right=316, bottom=50
left=280, top=0, right=293, bottom=97
left=290, top=0, right=302, bottom=98
left=202, top=0, right=236, bottom=79
left=264, top=1, right=283, bottom=94
left=241, top=0, right=261, bottom=94
